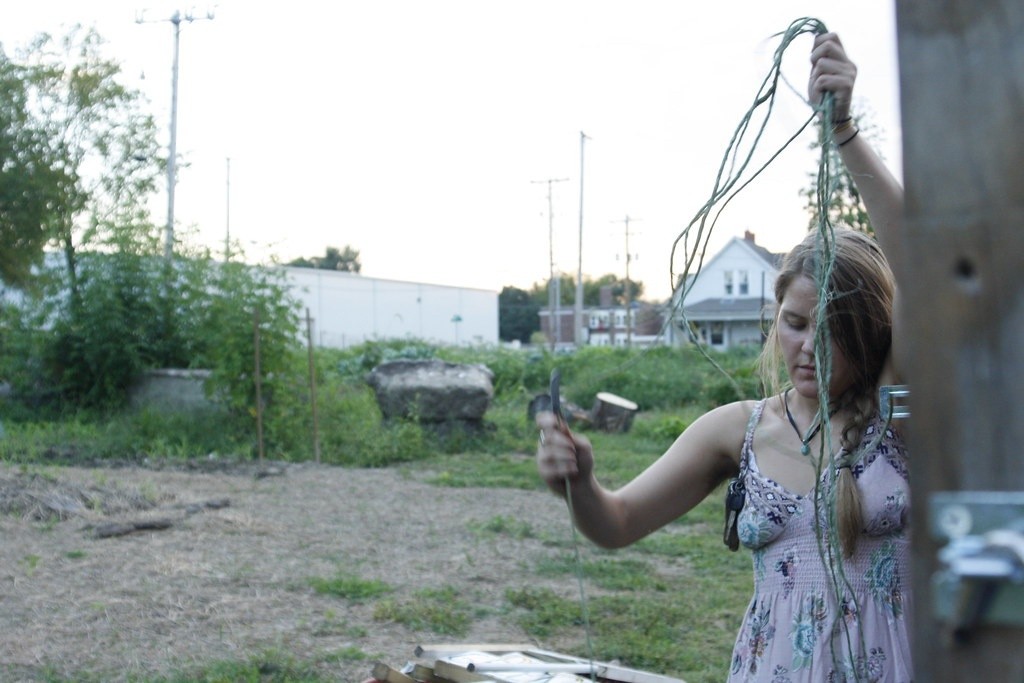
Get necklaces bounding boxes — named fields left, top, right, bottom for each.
left=784, top=388, right=842, bottom=456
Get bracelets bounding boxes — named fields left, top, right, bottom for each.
left=836, top=129, right=859, bottom=149
left=817, top=116, right=852, bottom=124
left=834, top=123, right=851, bottom=134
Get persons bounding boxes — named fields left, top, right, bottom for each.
left=535, top=33, right=915, bottom=683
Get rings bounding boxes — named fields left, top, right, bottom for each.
left=540, top=430, right=545, bottom=445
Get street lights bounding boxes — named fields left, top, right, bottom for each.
left=132, top=3, right=217, bottom=262
